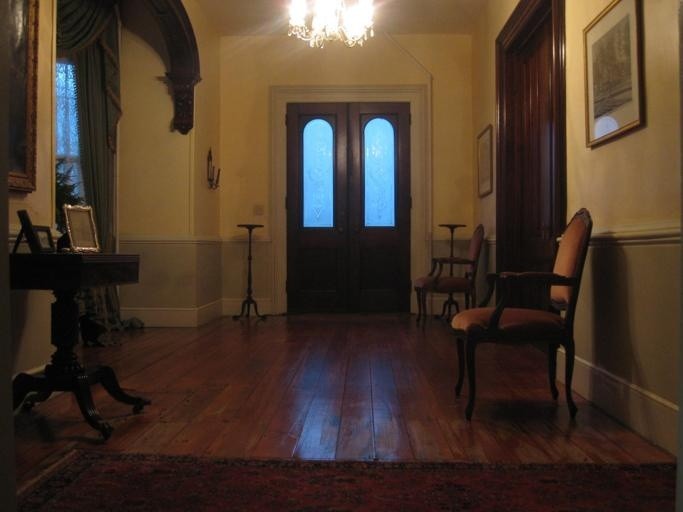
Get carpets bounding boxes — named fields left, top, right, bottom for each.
left=18, top=449, right=675, bottom=511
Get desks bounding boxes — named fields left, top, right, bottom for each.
left=8, top=254, right=153, bottom=441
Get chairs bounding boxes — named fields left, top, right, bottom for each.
left=451, top=207, right=593, bottom=421
left=414, top=224, right=484, bottom=324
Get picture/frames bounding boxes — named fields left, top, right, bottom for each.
left=18, top=209, right=56, bottom=253
left=63, top=203, right=102, bottom=254
left=7, top=0, right=39, bottom=193
left=477, top=124, right=493, bottom=198
left=583, top=0, right=643, bottom=147
